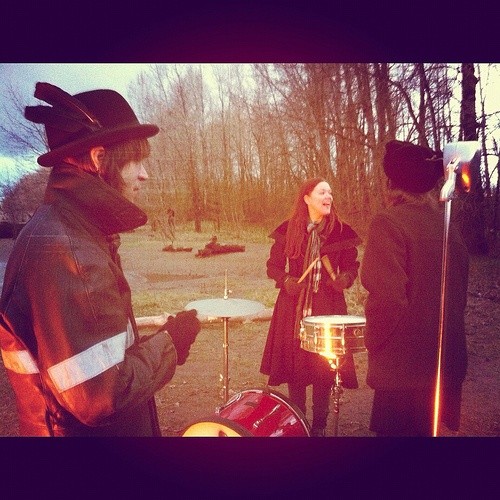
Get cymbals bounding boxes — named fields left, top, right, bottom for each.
left=184, top=298, right=264, bottom=317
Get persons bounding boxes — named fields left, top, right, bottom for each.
left=359, top=139, right=469, bottom=439
left=260, top=176, right=363, bottom=438
left=0, top=90, right=204, bottom=442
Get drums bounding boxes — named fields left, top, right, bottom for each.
left=179, top=388, right=313, bottom=437
left=299, top=315, right=368, bottom=354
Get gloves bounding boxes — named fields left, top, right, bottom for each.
left=161, top=308, right=200, bottom=353
left=141, top=329, right=190, bottom=365
left=282, top=275, right=307, bottom=296
left=326, top=273, right=350, bottom=291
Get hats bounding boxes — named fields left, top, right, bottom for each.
left=26, top=82, right=159, bottom=160
left=384, top=140, right=445, bottom=191
